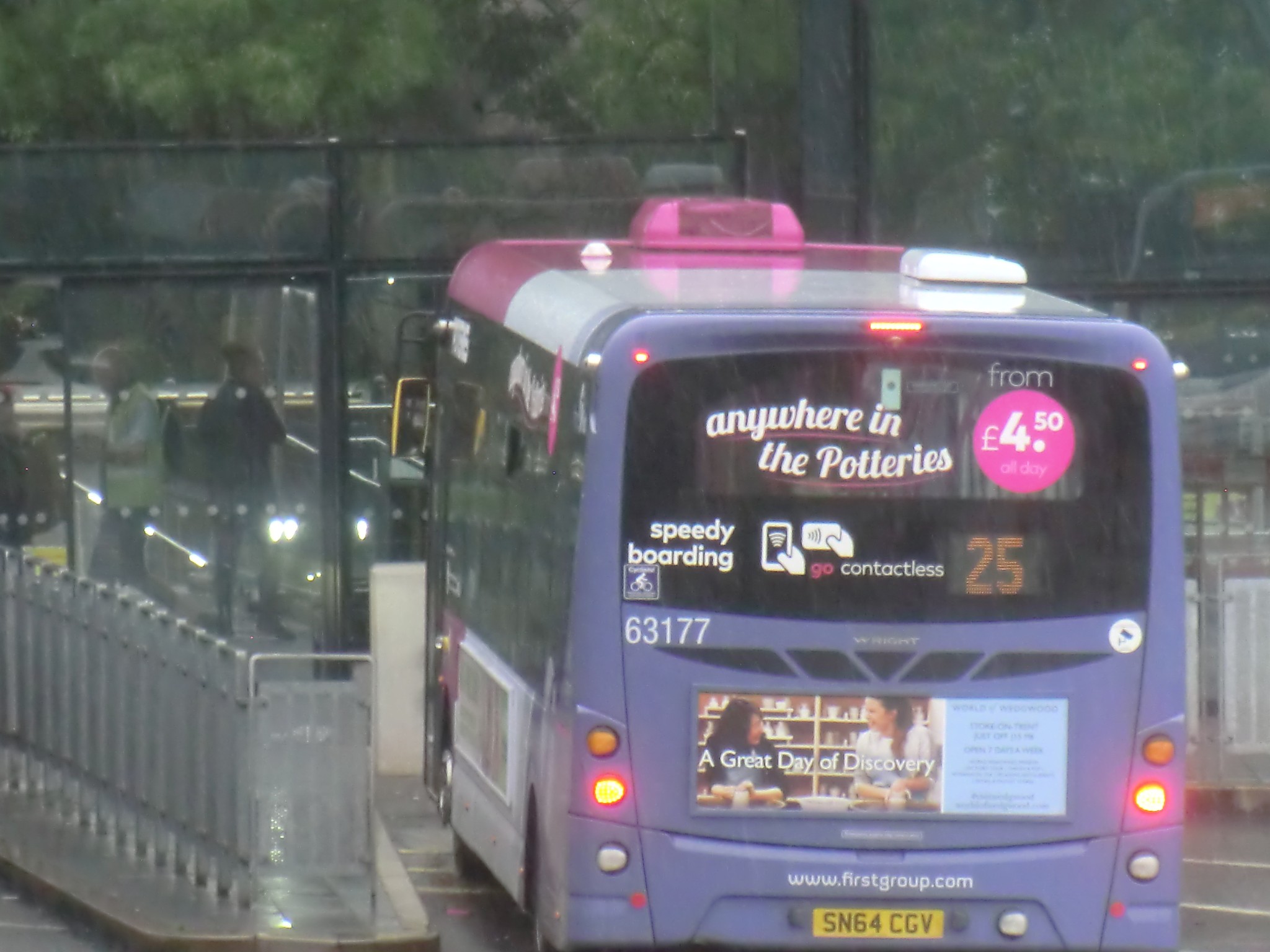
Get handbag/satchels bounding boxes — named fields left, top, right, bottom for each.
left=155, top=398, right=187, bottom=471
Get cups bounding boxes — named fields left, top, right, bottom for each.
left=818, top=728, right=857, bottom=798
left=761, top=697, right=865, bottom=720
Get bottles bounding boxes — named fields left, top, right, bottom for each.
left=704, top=720, right=715, bottom=742
left=763, top=720, right=788, bottom=737
left=706, top=695, right=730, bottom=708
left=914, top=706, right=924, bottom=727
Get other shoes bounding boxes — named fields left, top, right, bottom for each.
left=256, top=610, right=296, bottom=640
left=200, top=609, right=235, bottom=640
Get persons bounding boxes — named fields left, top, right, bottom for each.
left=86, top=344, right=166, bottom=592
left=193, top=338, right=298, bottom=641
left=702, top=696, right=793, bottom=798
left=851, top=697, right=937, bottom=804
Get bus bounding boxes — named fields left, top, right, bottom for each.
left=388, top=191, right=1192, bottom=951
